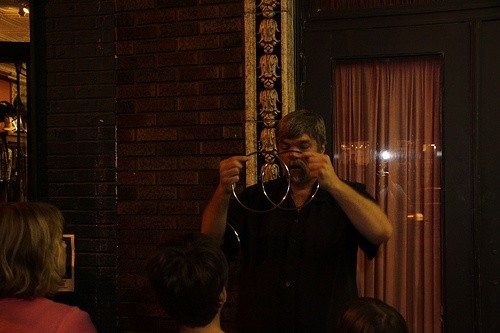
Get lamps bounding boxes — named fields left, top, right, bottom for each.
left=18, top=3, right=28, bottom=17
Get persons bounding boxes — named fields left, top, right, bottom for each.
left=200, top=109, right=390, bottom=333
left=335, top=297, right=408, bottom=333
left=0, top=201, right=96, bottom=333
left=149, top=233, right=233, bottom=333
